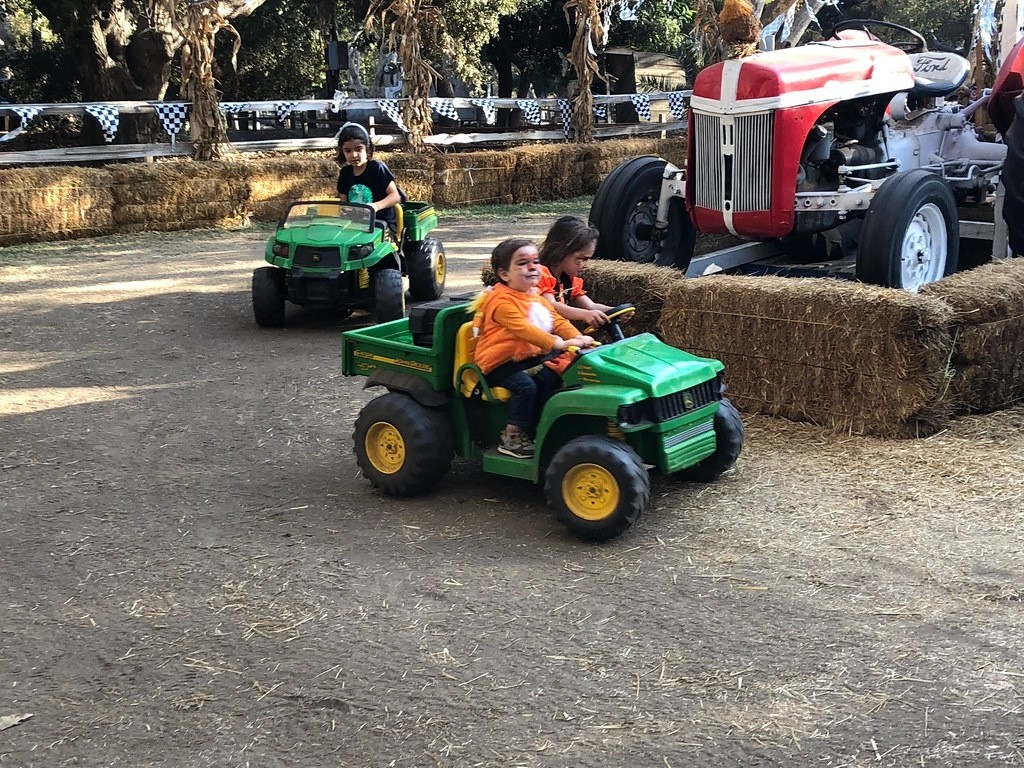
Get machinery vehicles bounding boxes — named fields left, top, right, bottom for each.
left=587, top=18, right=1024, bottom=293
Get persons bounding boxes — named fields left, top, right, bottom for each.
left=334, top=121, right=400, bottom=234
left=536, top=214, right=635, bottom=373
left=465, top=237, right=594, bottom=458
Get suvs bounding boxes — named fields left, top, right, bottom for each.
left=252, top=198, right=448, bottom=327
left=339, top=287, right=747, bottom=543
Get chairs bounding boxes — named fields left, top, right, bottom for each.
left=904, top=52, right=972, bottom=95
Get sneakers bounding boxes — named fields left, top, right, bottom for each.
left=497, top=430, right=536, bottom=458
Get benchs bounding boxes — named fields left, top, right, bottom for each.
left=454, top=321, right=513, bottom=403
left=308, top=197, right=404, bottom=251
